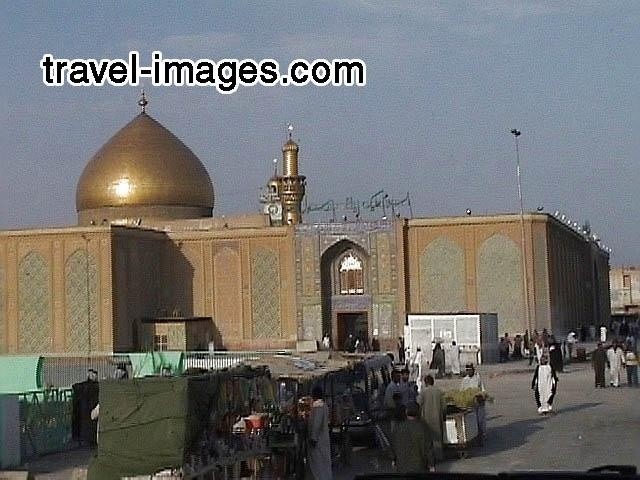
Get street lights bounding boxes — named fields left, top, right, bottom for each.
left=511, top=129, right=532, bottom=341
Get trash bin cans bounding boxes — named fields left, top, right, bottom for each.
left=577, top=347, right=586, bottom=362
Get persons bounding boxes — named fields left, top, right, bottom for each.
left=306, top=315, right=640, bottom=480
left=113, top=365, right=125, bottom=380
left=91, top=400, right=100, bottom=458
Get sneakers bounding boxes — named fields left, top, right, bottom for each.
left=594, top=381, right=638, bottom=388
left=539, top=410, right=554, bottom=417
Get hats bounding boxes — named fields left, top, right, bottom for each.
left=465, top=361, right=474, bottom=370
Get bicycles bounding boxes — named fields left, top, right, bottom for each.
left=329, top=425, right=352, bottom=472
